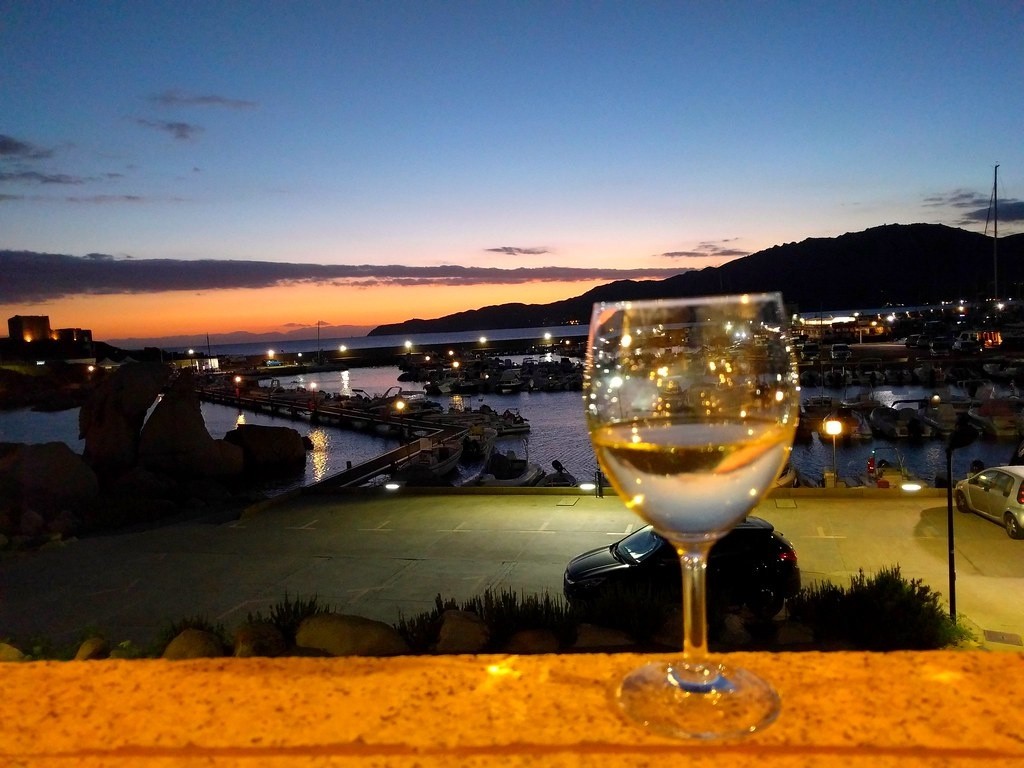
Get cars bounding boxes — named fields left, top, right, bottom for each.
left=955, top=466, right=1024, bottom=538
left=801, top=343, right=822, bottom=361
left=904, top=334, right=919, bottom=348
left=917, top=335, right=930, bottom=347
left=564, top=516, right=801, bottom=621
left=829, top=344, right=854, bottom=362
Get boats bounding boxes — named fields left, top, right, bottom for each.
left=248, top=379, right=577, bottom=488
left=857, top=448, right=930, bottom=488
left=800, top=299, right=1024, bottom=444
left=408, top=350, right=585, bottom=395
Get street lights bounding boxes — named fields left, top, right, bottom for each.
left=946, top=424, right=978, bottom=625
left=311, top=382, right=316, bottom=412
left=825, top=420, right=843, bottom=487
left=396, top=402, right=406, bottom=447
left=188, top=349, right=195, bottom=376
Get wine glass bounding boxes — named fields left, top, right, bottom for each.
left=584, top=289, right=804, bottom=746
